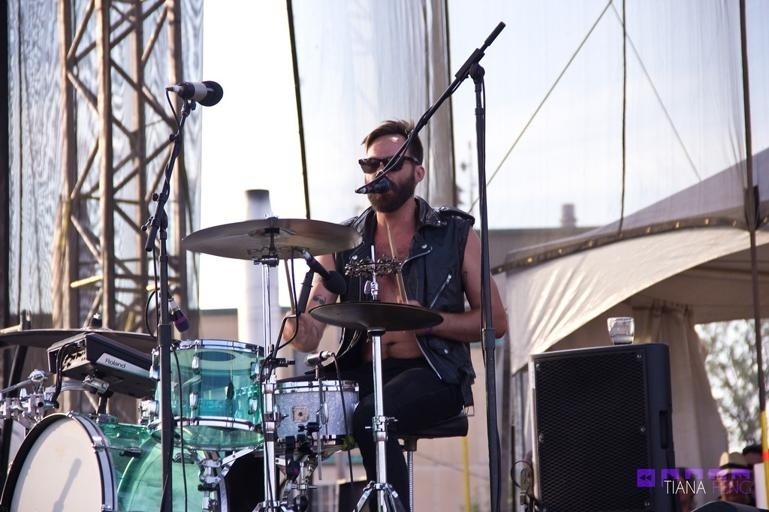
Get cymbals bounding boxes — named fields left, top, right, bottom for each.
left=1, top=326, right=180, bottom=353
left=182, top=217, right=362, bottom=260
left=308, top=300, right=444, bottom=331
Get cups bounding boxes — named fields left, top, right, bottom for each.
left=607, top=315, right=636, bottom=345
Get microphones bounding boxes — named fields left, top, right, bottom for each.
left=296, top=247, right=346, bottom=295
left=304, top=350, right=332, bottom=366
left=167, top=81, right=223, bottom=107
left=167, top=293, right=189, bottom=332
left=355, top=179, right=389, bottom=193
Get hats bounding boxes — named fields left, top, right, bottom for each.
left=717, top=452, right=749, bottom=476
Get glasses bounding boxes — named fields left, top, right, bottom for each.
left=359, top=156, right=420, bottom=173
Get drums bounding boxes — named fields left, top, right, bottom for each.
left=272, top=379, right=360, bottom=453
left=2, top=410, right=231, bottom=512
left=146, top=338, right=265, bottom=451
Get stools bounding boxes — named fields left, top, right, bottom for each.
left=399, top=414, right=468, bottom=511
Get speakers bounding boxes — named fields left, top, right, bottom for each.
left=529, top=343, right=680, bottom=512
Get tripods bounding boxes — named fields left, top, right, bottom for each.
left=351, top=334, right=406, bottom=512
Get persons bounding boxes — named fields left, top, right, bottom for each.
left=714, top=444, right=763, bottom=508
left=218, top=119, right=508, bottom=511
